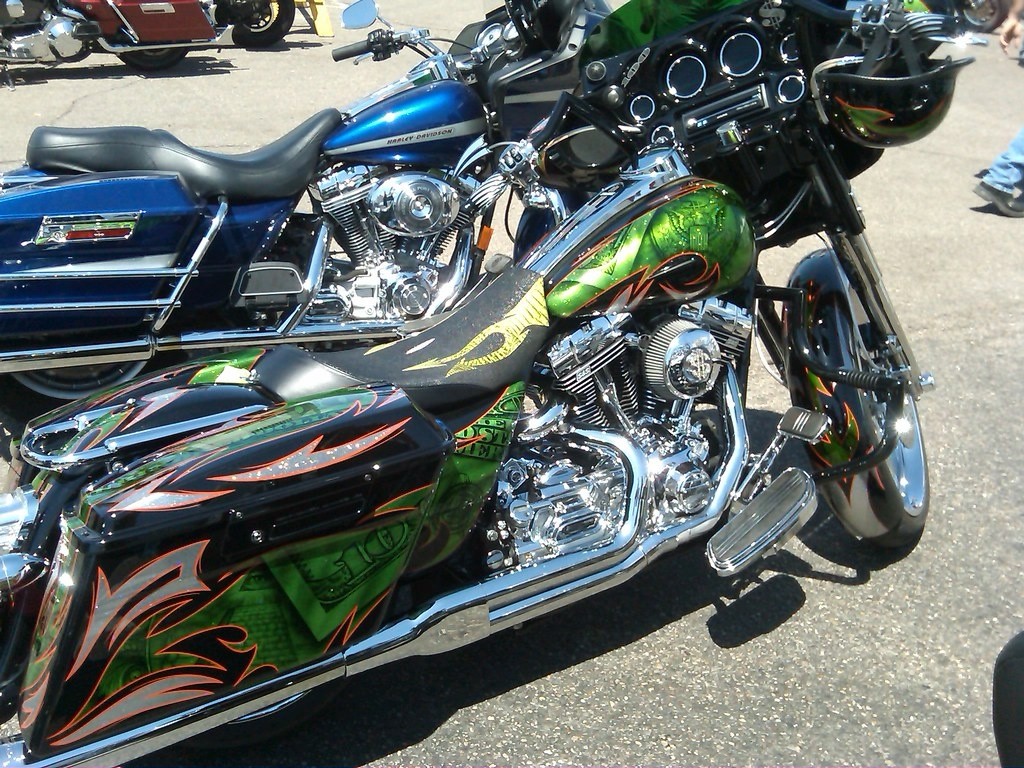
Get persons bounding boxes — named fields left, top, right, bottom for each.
left=973, top=0, right=1024, bottom=217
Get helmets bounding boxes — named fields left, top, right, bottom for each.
left=821, top=12, right=975, bottom=149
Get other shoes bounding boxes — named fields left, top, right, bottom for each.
left=973, top=182, right=1024, bottom=218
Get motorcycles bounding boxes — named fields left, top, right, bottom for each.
left=0, top=0, right=975, bottom=768
left=0, top=0, right=296, bottom=91
left=0, top=0, right=639, bottom=423
